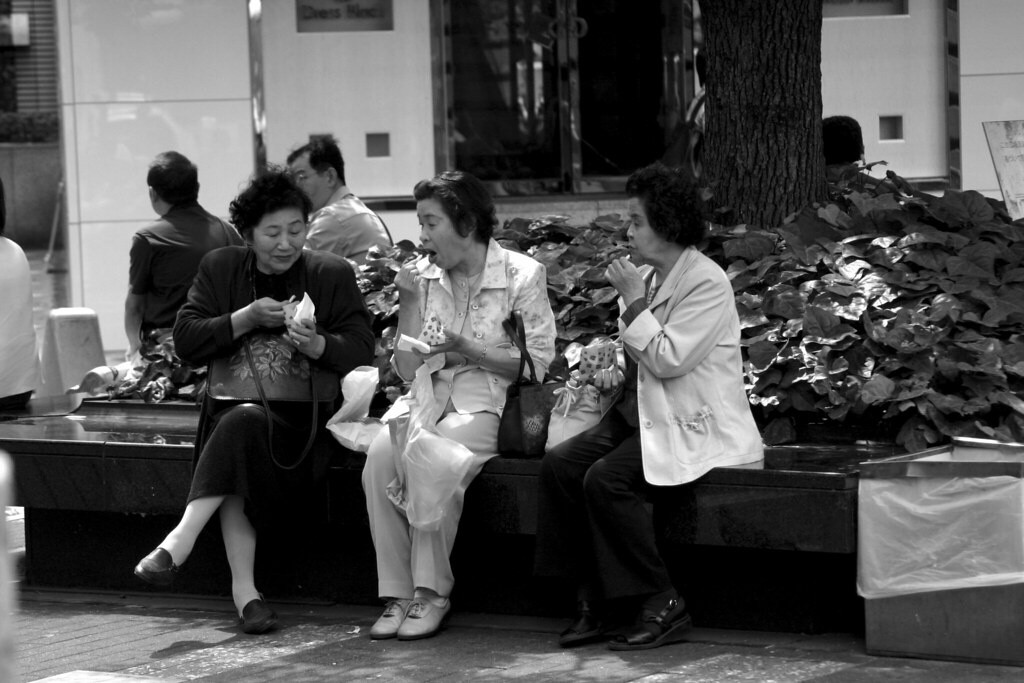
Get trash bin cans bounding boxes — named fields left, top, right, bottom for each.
left=860, top=448, right=1023, bottom=666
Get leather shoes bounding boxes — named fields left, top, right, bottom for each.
left=133, top=548, right=185, bottom=582
left=238, top=591, right=279, bottom=632
left=368, top=598, right=410, bottom=640
left=397, top=595, right=451, bottom=640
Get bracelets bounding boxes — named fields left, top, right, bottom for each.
left=475, top=344, right=488, bottom=365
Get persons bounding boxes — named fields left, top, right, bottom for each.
left=134, top=172, right=375, bottom=633
left=285, top=134, right=394, bottom=265
left=125, top=151, right=245, bottom=362
left=0, top=180, right=36, bottom=414
left=539, top=162, right=765, bottom=649
left=362, top=171, right=558, bottom=640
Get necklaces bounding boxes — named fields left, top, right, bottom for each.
left=450, top=266, right=482, bottom=298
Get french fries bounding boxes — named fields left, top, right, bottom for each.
left=289, top=294, right=296, bottom=303
left=416, top=254, right=423, bottom=261
left=624, top=254, right=630, bottom=261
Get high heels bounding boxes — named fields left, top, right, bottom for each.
left=609, top=590, right=693, bottom=650
left=559, top=593, right=621, bottom=644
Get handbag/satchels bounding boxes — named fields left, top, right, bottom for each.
left=205, top=325, right=336, bottom=413
left=499, top=309, right=569, bottom=456
left=547, top=377, right=610, bottom=453
